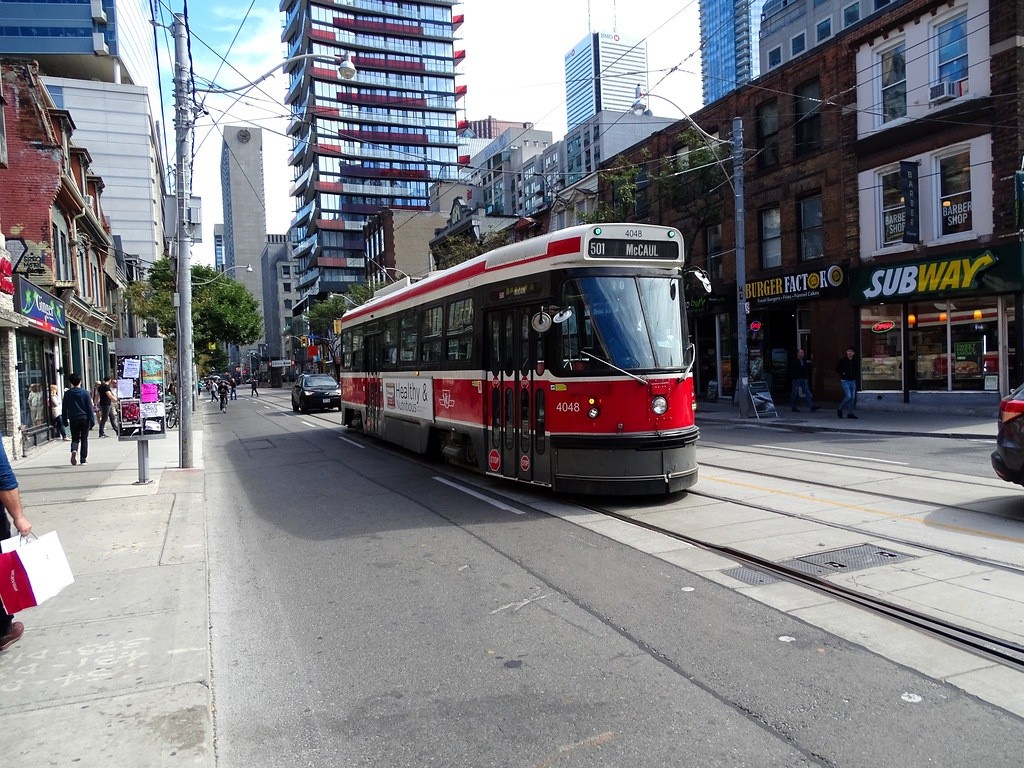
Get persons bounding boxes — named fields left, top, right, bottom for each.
left=838, top=348, right=859, bottom=419
left=790, top=350, right=820, bottom=412
left=62, top=373, right=94, bottom=466
left=210, top=380, right=218, bottom=403
left=230, top=378, right=237, bottom=400
left=29, top=383, right=43, bottom=427
left=0, top=434, right=31, bottom=650
left=97, top=376, right=119, bottom=438
left=50, top=385, right=73, bottom=441
left=218, top=381, right=230, bottom=411
left=250, top=379, right=259, bottom=397
left=199, top=381, right=203, bottom=395
left=94, top=381, right=106, bottom=430
left=109, top=380, right=120, bottom=415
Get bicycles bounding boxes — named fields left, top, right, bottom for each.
left=167, top=402, right=179, bottom=429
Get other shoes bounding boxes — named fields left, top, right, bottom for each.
left=838, top=409, right=843, bottom=419
left=64, top=437, right=71, bottom=441
left=80, top=460, right=87, bottom=464
left=71, top=450, right=77, bottom=465
left=0, top=622, right=24, bottom=649
left=99, top=434, right=109, bottom=437
left=792, top=408, right=800, bottom=412
left=847, top=413, right=858, bottom=419
left=811, top=405, right=821, bottom=411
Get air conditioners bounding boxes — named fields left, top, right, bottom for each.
left=928, top=82, right=959, bottom=104
left=83, top=195, right=94, bottom=208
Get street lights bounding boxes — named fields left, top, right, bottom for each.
left=173, top=50, right=355, bottom=469
left=632, top=83, right=752, bottom=418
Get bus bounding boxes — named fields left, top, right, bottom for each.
left=341, top=173, right=712, bottom=495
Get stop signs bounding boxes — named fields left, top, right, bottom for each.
left=489, top=449, right=500, bottom=471
left=521, top=456, right=529, bottom=471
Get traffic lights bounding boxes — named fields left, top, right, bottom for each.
left=301, top=337, right=308, bottom=348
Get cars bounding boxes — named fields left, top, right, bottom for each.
left=292, top=374, right=341, bottom=413
left=991, top=383, right=1024, bottom=487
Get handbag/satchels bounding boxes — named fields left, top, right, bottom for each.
left=0, top=530, right=74, bottom=615
